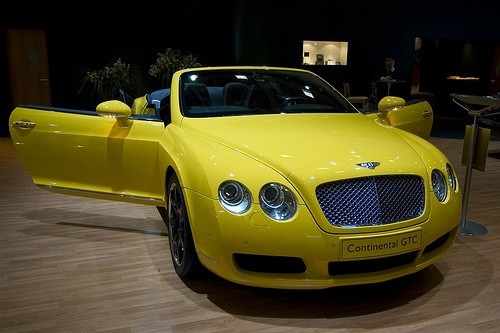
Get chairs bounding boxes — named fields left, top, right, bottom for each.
left=344, top=82, right=368, bottom=108
left=180, top=81, right=213, bottom=108
left=245, top=82, right=280, bottom=110
left=222, top=82, right=250, bottom=106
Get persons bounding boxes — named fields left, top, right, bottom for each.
left=376, top=54, right=401, bottom=110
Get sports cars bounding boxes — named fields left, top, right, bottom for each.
left=8, top=64, right=463, bottom=291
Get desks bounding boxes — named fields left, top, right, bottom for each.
left=374, top=78, right=408, bottom=96
